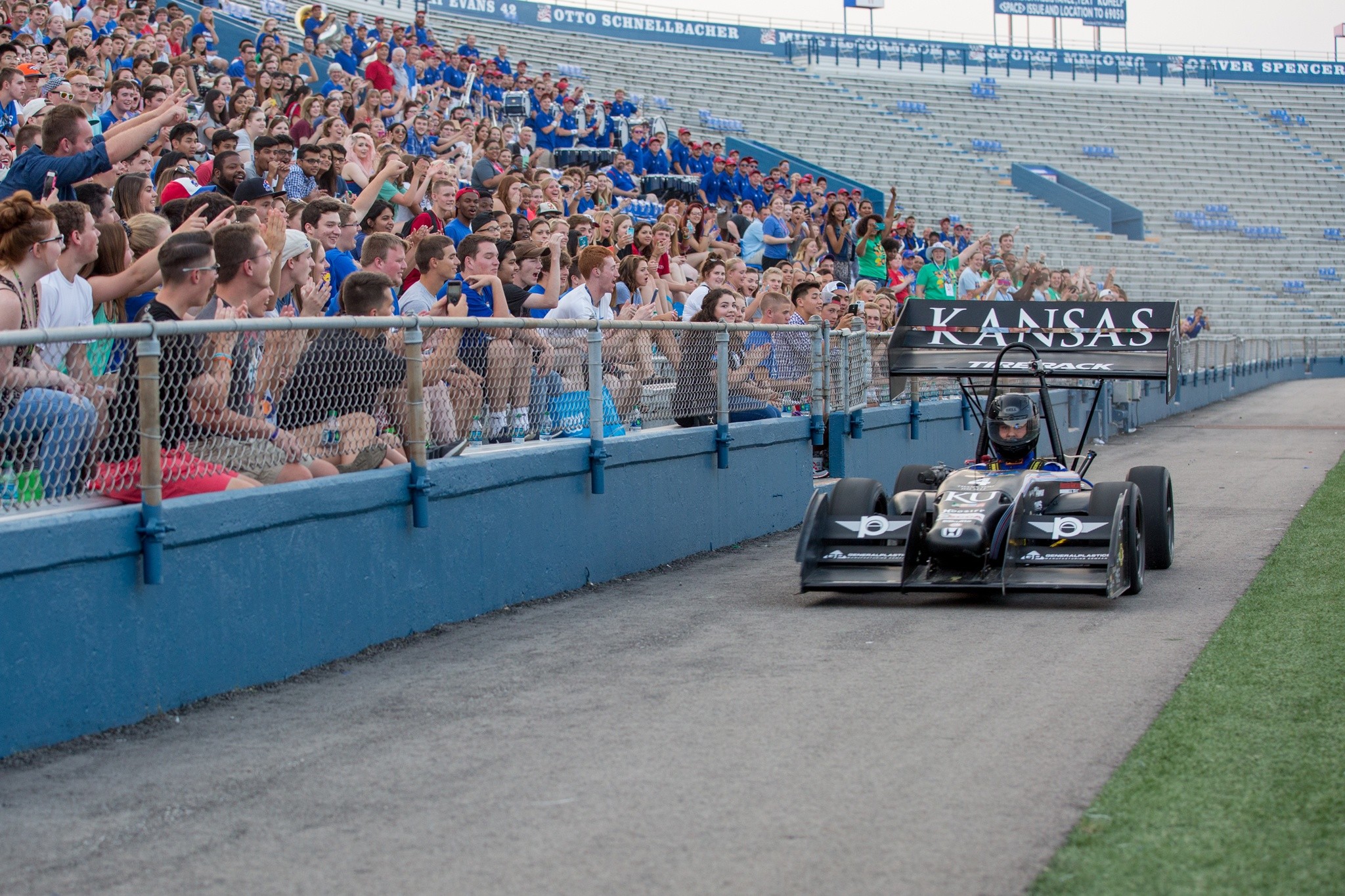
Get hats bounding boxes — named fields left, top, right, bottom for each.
left=821, top=280, right=849, bottom=304
left=890, top=217, right=1007, bottom=277
left=513, top=239, right=551, bottom=262
left=1098, top=289, right=1114, bottom=298
left=280, top=229, right=311, bottom=270
left=818, top=253, right=834, bottom=265
left=310, top=4, right=862, bottom=219
left=42, top=77, right=72, bottom=98
left=471, top=210, right=498, bottom=234
left=234, top=178, right=288, bottom=204
left=583, top=213, right=600, bottom=228
left=16, top=63, right=48, bottom=79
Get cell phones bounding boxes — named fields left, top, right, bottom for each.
left=1199, top=315, right=1206, bottom=320
left=421, top=103, right=430, bottom=112
left=1069, top=287, right=1076, bottom=294
left=804, top=208, right=811, bottom=217
left=845, top=218, right=851, bottom=226
left=579, top=236, right=588, bottom=249
left=651, top=288, right=658, bottom=304
left=41, top=169, right=58, bottom=203
left=585, top=181, right=591, bottom=194
left=445, top=280, right=463, bottom=315
left=856, top=301, right=865, bottom=314
left=847, top=304, right=858, bottom=316
left=627, top=228, right=634, bottom=237
left=75, top=61, right=82, bottom=69
left=48, top=52, right=56, bottom=60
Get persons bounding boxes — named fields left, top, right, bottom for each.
left=0, top=0, right=1207, bottom=495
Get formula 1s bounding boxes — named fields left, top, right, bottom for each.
left=794, top=296, right=1186, bottom=594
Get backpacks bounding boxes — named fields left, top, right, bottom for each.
left=402, top=208, right=438, bottom=238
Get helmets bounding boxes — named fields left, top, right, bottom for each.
left=986, top=392, right=1041, bottom=459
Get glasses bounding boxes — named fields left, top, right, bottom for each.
left=28, top=233, right=64, bottom=253
left=301, top=158, right=322, bottom=165
left=279, top=150, right=295, bottom=157
left=478, top=226, right=502, bottom=234
left=70, top=83, right=105, bottom=92
left=345, top=221, right=360, bottom=227
left=181, top=263, right=220, bottom=274
left=237, top=249, right=271, bottom=264
left=50, top=91, right=75, bottom=101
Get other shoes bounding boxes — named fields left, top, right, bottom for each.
left=336, top=424, right=565, bottom=473
